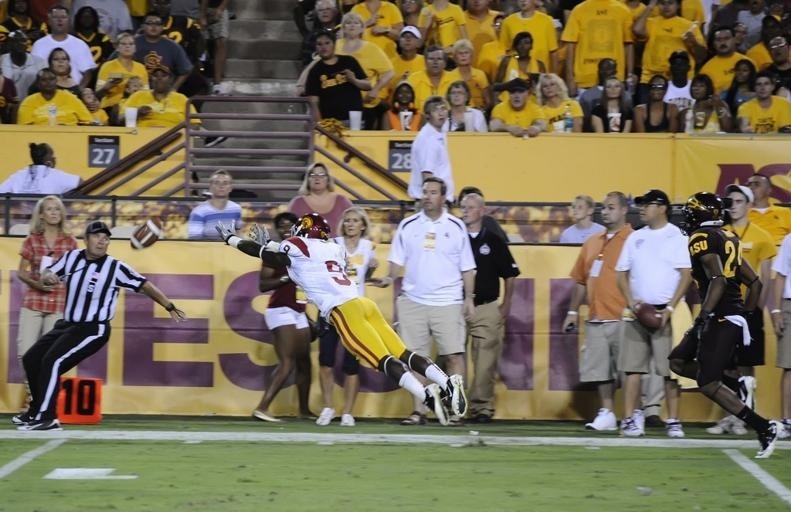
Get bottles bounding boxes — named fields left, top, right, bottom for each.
left=46, top=102, right=58, bottom=128
left=683, top=107, right=696, bottom=137
left=680, top=18, right=701, bottom=41
left=563, top=105, right=575, bottom=136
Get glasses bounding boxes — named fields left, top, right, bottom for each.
left=492, top=22, right=502, bottom=29
left=647, top=84, right=667, bottom=91
left=143, top=21, right=163, bottom=26
left=713, top=36, right=734, bottom=41
left=308, top=172, right=328, bottom=178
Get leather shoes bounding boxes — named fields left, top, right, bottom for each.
left=253, top=409, right=282, bottom=422
left=477, top=414, right=490, bottom=423
left=298, top=412, right=319, bottom=420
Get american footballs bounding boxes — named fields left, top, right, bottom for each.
left=633, top=302, right=661, bottom=329
left=130, top=217, right=162, bottom=250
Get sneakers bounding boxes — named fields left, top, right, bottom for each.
left=729, top=421, right=748, bottom=436
left=422, top=383, right=449, bottom=426
left=204, top=135, right=229, bottom=147
left=705, top=421, right=731, bottom=434
left=663, top=418, right=685, bottom=438
left=400, top=411, right=428, bottom=425
left=646, top=415, right=665, bottom=427
left=11, top=412, right=39, bottom=425
left=447, top=419, right=465, bottom=426
left=584, top=407, right=618, bottom=430
left=754, top=419, right=784, bottom=458
left=620, top=417, right=645, bottom=437
left=211, top=83, right=233, bottom=97
left=632, top=408, right=646, bottom=431
left=444, top=374, right=469, bottom=417
left=780, top=418, right=791, bottom=432
left=316, top=407, right=337, bottom=426
left=16, top=418, right=63, bottom=431
left=341, top=414, right=355, bottom=427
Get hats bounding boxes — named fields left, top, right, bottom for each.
left=85, top=221, right=112, bottom=237
left=724, top=183, right=754, bottom=203
left=399, top=25, right=422, bottom=39
left=507, top=77, right=528, bottom=90
left=668, top=50, right=689, bottom=64
left=151, top=64, right=172, bottom=75
left=634, top=190, right=669, bottom=205
left=761, top=14, right=784, bottom=28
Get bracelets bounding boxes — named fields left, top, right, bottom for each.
left=770, top=308, right=782, bottom=314
left=567, top=309, right=579, bottom=317
left=465, top=292, right=479, bottom=299
left=165, top=303, right=176, bottom=313
left=666, top=305, right=679, bottom=316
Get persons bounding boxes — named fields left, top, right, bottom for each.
left=74, top=6, right=115, bottom=65
left=250, top=213, right=320, bottom=423
left=704, top=1, right=720, bottom=25
left=705, top=183, right=777, bottom=437
left=189, top=169, right=247, bottom=242
left=416, top=0, right=463, bottom=47
left=307, top=29, right=372, bottom=128
left=700, top=26, right=755, bottom=91
left=536, top=73, right=572, bottom=133
left=11, top=196, right=78, bottom=408
left=408, top=46, right=458, bottom=104
left=147, top=0, right=205, bottom=61
left=591, top=75, right=634, bottom=134
left=635, top=74, right=677, bottom=134
left=719, top=59, right=758, bottom=105
left=0, top=142, right=83, bottom=197
left=449, top=39, right=493, bottom=112
left=447, top=80, right=487, bottom=132
left=303, top=0, right=343, bottom=37
left=390, top=78, right=415, bottom=130
left=0, top=65, right=19, bottom=123
left=736, top=76, right=791, bottom=135
left=132, top=11, right=193, bottom=91
left=493, top=32, right=546, bottom=83
left=407, top=96, right=455, bottom=204
left=745, top=173, right=791, bottom=238
left=499, top=0, right=563, bottom=77
left=681, top=75, right=732, bottom=133
left=79, top=86, right=109, bottom=126
left=561, top=1, right=633, bottom=98
left=13, top=220, right=187, bottom=432
left=458, top=187, right=511, bottom=247
left=560, top=193, right=606, bottom=242
left=618, top=190, right=693, bottom=435
left=760, top=30, right=791, bottom=98
left=398, top=0, right=424, bottom=29
left=726, top=22, right=751, bottom=50
left=562, top=190, right=637, bottom=432
left=639, top=350, right=668, bottom=427
left=1, top=31, right=32, bottom=82
left=762, top=1, right=782, bottom=42
left=389, top=26, right=427, bottom=89
left=457, top=193, right=520, bottom=424
left=28, top=6, right=98, bottom=95
left=737, top=0, right=766, bottom=50
left=667, top=51, right=692, bottom=131
left=115, top=66, right=201, bottom=130
left=316, top=206, right=380, bottom=427
left=770, top=232, right=791, bottom=439
left=291, top=162, right=353, bottom=237
left=462, top=0, right=500, bottom=43
left=48, top=46, right=80, bottom=95
left=216, top=213, right=469, bottom=424
left=146, top=1, right=208, bottom=77
left=94, top=33, right=149, bottom=123
left=377, top=178, right=477, bottom=426
left=490, top=78, right=548, bottom=138
left=315, top=12, right=394, bottom=131
left=667, top=185, right=784, bottom=460
left=345, top=2, right=402, bottom=37
left=118, top=76, right=144, bottom=122
left=200, top=1, right=229, bottom=84
left=631, top=0, right=706, bottom=81
left=15, top=67, right=98, bottom=126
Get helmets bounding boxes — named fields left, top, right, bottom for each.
left=677, top=192, right=725, bottom=236
left=289, top=212, right=330, bottom=241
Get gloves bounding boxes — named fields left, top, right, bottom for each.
left=214, top=219, right=236, bottom=245
left=745, top=310, right=756, bottom=328
left=248, top=223, right=271, bottom=246
left=685, top=317, right=706, bottom=342
left=562, top=311, right=579, bottom=334
